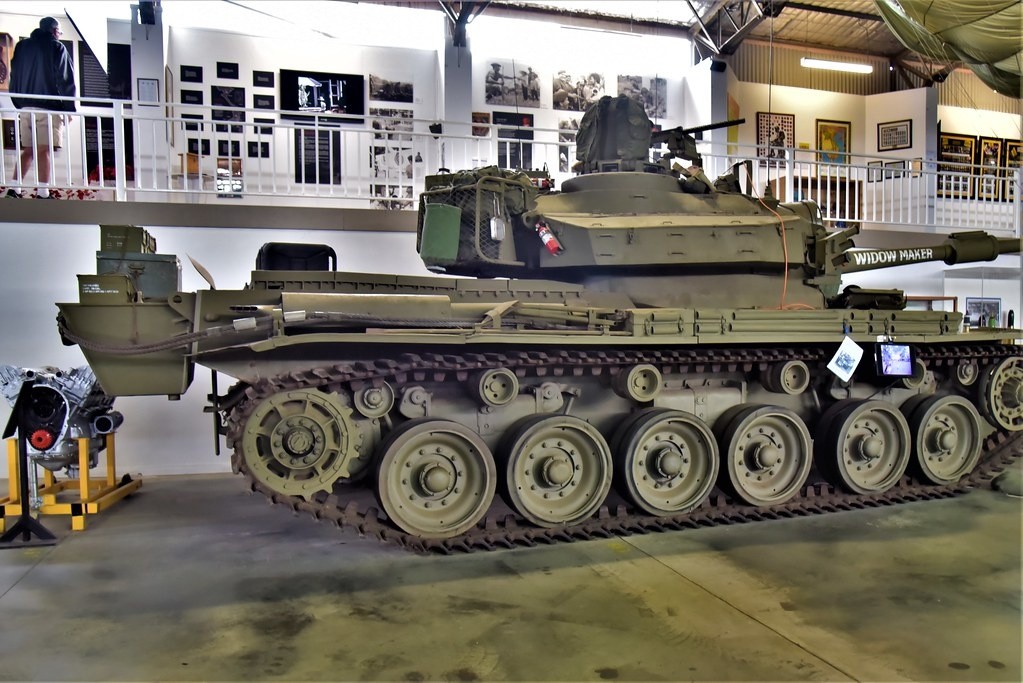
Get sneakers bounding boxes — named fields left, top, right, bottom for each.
left=4, top=189, right=23, bottom=199
left=37, top=193, right=55, bottom=199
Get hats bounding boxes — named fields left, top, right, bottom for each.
left=491, top=63, right=501, bottom=67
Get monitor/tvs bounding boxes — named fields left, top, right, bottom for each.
left=279, top=68, right=365, bottom=125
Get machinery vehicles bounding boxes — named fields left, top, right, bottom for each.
left=54, top=95, right=1023, bottom=555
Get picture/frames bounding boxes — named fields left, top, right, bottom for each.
left=908, top=158, right=922, bottom=177
left=966, top=297, right=1002, bottom=328
left=756, top=111, right=795, bottom=168
left=885, top=161, right=905, bottom=179
left=815, top=119, right=851, bottom=177
left=1003, top=139, right=1023, bottom=202
left=867, top=161, right=883, bottom=183
left=877, top=119, right=913, bottom=152
left=937, top=131, right=978, bottom=199
left=979, top=136, right=1003, bottom=201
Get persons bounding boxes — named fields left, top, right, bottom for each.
left=4, top=17, right=76, bottom=200
left=486, top=62, right=666, bottom=173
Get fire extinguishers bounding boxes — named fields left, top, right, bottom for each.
left=534, top=220, right=559, bottom=254
left=530, top=178, right=555, bottom=189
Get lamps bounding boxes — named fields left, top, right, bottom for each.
left=800, top=10, right=873, bottom=75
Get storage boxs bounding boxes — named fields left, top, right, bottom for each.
left=99, top=223, right=158, bottom=253
left=96, top=251, right=182, bottom=300
left=76, top=274, right=134, bottom=305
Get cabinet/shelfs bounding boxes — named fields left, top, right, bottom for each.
left=769, top=175, right=863, bottom=228
